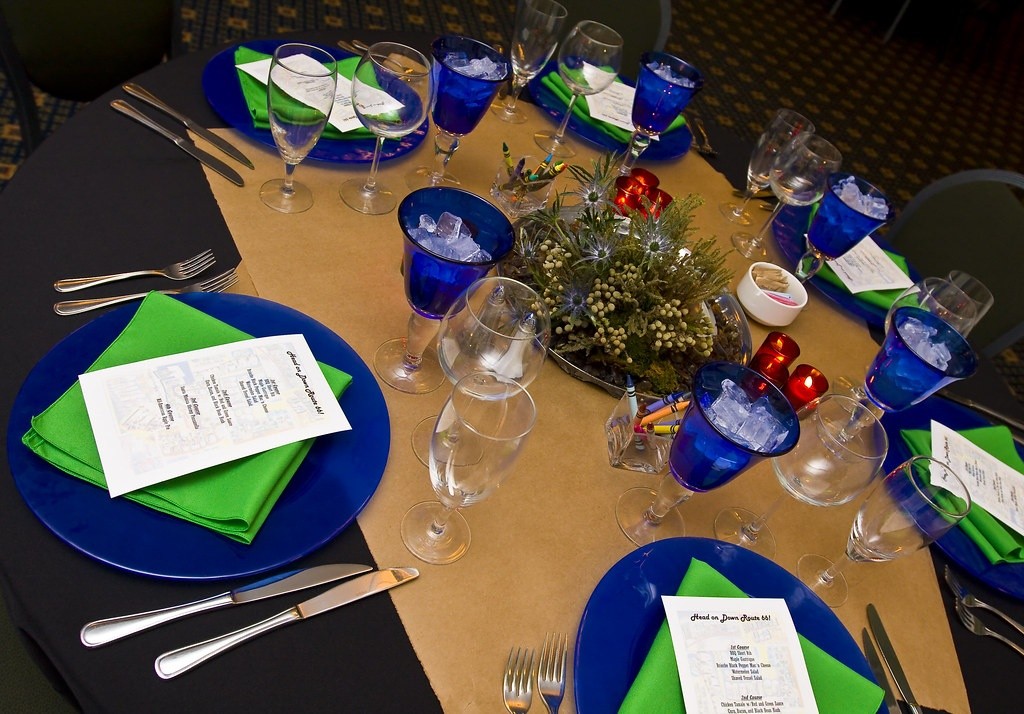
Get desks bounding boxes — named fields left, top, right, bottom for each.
left=0, top=24, right=1024, bottom=714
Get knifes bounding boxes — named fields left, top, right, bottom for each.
left=734, top=189, right=776, bottom=210
left=109, top=81, right=254, bottom=186
left=862, top=603, right=923, bottom=714
left=81, top=562, right=420, bottom=679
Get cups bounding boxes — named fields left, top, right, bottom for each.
left=779, top=364, right=830, bottom=413
left=609, top=168, right=674, bottom=223
left=740, top=354, right=789, bottom=403
left=747, top=331, right=800, bottom=369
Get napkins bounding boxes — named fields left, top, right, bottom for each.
left=801, top=192, right=932, bottom=317
left=19, top=287, right=354, bottom=545
left=618, top=558, right=886, bottom=714
left=541, top=66, right=688, bottom=144
left=899, top=423, right=1024, bottom=565
left=233, top=45, right=405, bottom=140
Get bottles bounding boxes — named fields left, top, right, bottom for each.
left=458, top=284, right=507, bottom=359
left=488, top=311, right=537, bottom=384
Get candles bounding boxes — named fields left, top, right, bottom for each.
left=611, top=167, right=673, bottom=222
left=741, top=331, right=829, bottom=421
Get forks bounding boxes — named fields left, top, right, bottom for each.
left=503, top=632, right=568, bottom=714
left=681, top=113, right=721, bottom=155
left=337, top=39, right=425, bottom=81
left=52, top=248, right=239, bottom=315
left=944, top=565, right=1024, bottom=655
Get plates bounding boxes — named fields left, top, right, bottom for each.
left=573, top=536, right=889, bottom=714
left=530, top=57, right=692, bottom=161
left=202, top=38, right=430, bottom=163
left=772, top=201, right=922, bottom=328
left=5, top=292, right=390, bottom=578
left=873, top=393, right=1024, bottom=600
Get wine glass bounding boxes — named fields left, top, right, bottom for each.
left=796, top=455, right=971, bottom=607
left=713, top=393, right=888, bottom=560
left=372, top=186, right=552, bottom=566
left=615, top=360, right=801, bottom=546
left=259, top=35, right=513, bottom=215
left=719, top=108, right=895, bottom=284
left=490, top=0, right=704, bottom=180
left=817, top=270, right=994, bottom=461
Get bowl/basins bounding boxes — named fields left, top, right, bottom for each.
left=737, top=261, right=808, bottom=327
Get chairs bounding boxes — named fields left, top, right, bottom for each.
left=0, top=0, right=187, bottom=165
left=877, top=169, right=1024, bottom=360
left=510, top=0, right=673, bottom=82
